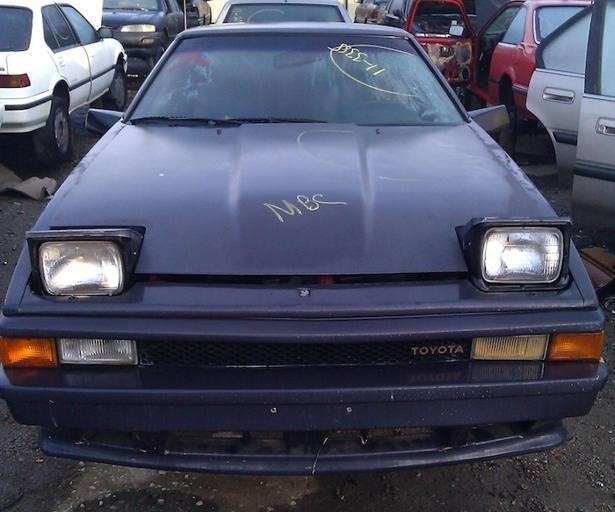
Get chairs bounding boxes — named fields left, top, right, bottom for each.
left=323, top=53, right=377, bottom=120
left=193, top=63, right=266, bottom=116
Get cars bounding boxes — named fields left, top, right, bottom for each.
left=0, top=18, right=607, bottom=477
left=102, top=0, right=184, bottom=91
left=178, top=0, right=212, bottom=27
left=215, top=0, right=355, bottom=29
left=1, top=1, right=128, bottom=169
